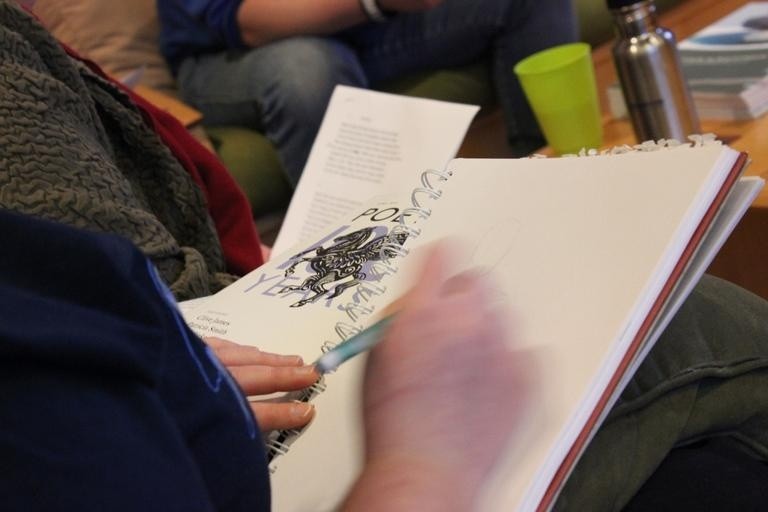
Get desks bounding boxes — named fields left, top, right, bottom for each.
left=521, top=1, right=767, bottom=299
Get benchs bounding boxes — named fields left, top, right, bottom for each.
left=126, top=1, right=680, bottom=218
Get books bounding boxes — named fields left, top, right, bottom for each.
left=606, top=1, right=768, bottom=124
left=269, top=131, right=764, bottom=512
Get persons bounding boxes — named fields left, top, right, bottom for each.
left=155, top=0, right=576, bottom=194
left=0, top=211, right=768, bottom=512
left=0, top=0, right=271, bottom=303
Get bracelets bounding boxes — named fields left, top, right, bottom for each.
left=362, top=0, right=384, bottom=22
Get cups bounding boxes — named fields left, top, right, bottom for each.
left=511, top=40, right=604, bottom=159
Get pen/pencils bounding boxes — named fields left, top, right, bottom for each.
left=309, top=315, right=398, bottom=375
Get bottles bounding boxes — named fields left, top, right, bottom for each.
left=602, top=0, right=704, bottom=147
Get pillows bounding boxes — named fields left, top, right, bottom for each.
left=554, top=272, right=768, bottom=511
